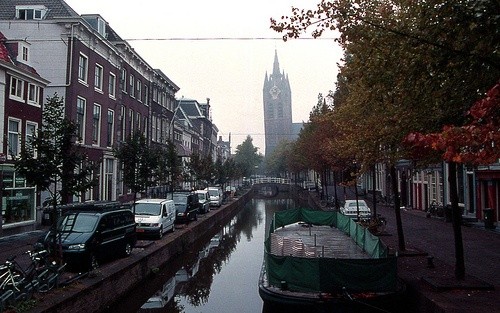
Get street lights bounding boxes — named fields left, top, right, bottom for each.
left=352, top=159, right=360, bottom=222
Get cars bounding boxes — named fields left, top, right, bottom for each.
left=339, top=199, right=372, bottom=222
left=303, top=181, right=317, bottom=190
left=223, top=180, right=250, bottom=200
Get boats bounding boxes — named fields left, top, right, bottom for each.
left=258, top=203, right=407, bottom=313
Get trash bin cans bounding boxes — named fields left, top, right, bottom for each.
left=41, top=200, right=61, bottom=226
left=484, top=208, right=497, bottom=229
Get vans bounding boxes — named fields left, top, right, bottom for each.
left=43, top=207, right=137, bottom=270
left=163, top=191, right=200, bottom=225
left=130, top=198, right=176, bottom=239
left=203, top=186, right=224, bottom=208
left=190, top=189, right=211, bottom=214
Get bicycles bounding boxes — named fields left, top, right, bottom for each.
left=0, top=241, right=58, bottom=313
left=366, top=211, right=387, bottom=236
